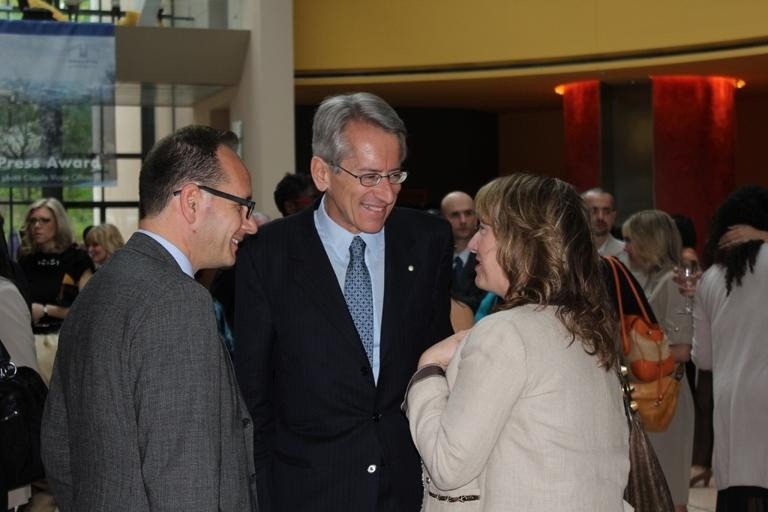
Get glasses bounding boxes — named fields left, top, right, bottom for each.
left=337, top=164, right=409, bottom=188
left=173, top=185, right=256, bottom=221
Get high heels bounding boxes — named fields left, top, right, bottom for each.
left=689, top=469, right=713, bottom=486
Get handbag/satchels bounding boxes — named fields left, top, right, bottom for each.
left=0, top=337, right=50, bottom=494
left=604, top=255, right=680, bottom=434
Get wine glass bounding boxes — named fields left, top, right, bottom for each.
left=674, top=259, right=698, bottom=315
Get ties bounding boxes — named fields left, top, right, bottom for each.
left=454, top=255, right=464, bottom=291
left=343, top=236, right=374, bottom=372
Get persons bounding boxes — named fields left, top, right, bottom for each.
left=38, top=123, right=260, bottom=511
left=219, top=92, right=461, bottom=510
left=689, top=182, right=768, bottom=512
